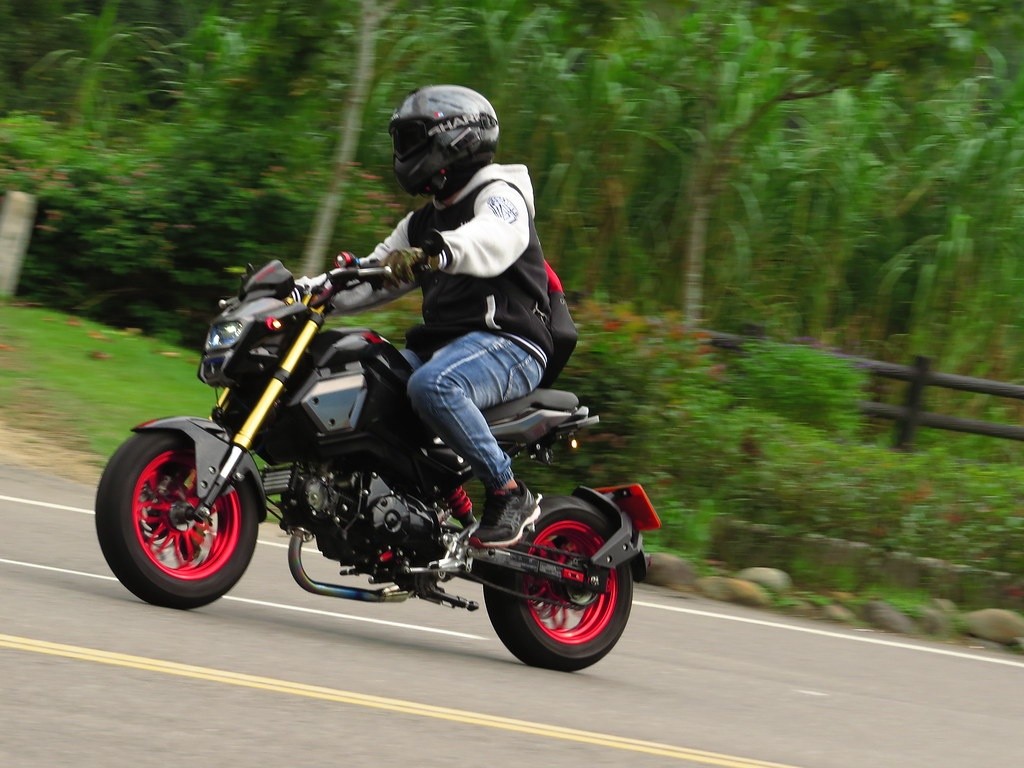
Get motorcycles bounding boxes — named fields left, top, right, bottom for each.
left=94, top=251, right=662, bottom=672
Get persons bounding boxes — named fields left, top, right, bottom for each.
left=289, top=84, right=553, bottom=546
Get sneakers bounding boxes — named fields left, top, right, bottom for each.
left=469, top=478, right=541, bottom=549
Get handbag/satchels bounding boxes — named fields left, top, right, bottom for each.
left=538, top=258, right=578, bottom=389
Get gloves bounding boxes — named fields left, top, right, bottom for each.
left=384, top=248, right=440, bottom=288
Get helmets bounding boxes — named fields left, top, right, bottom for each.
left=388, top=84, right=500, bottom=201
